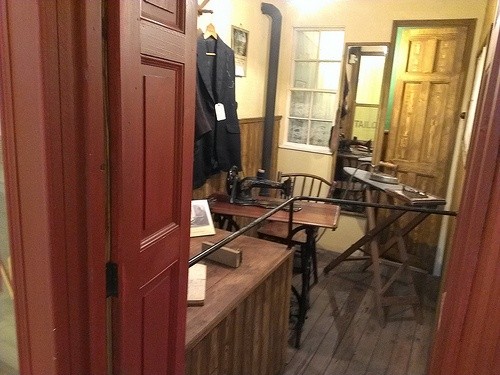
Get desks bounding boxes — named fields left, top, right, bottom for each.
left=324, top=167, right=447, bottom=329
left=202, top=193, right=340, bottom=351
left=183, top=227, right=296, bottom=375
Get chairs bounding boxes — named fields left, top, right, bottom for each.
left=255, top=170, right=335, bottom=285
left=332, top=162, right=373, bottom=211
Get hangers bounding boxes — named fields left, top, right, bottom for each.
left=196, top=10, right=234, bottom=56
